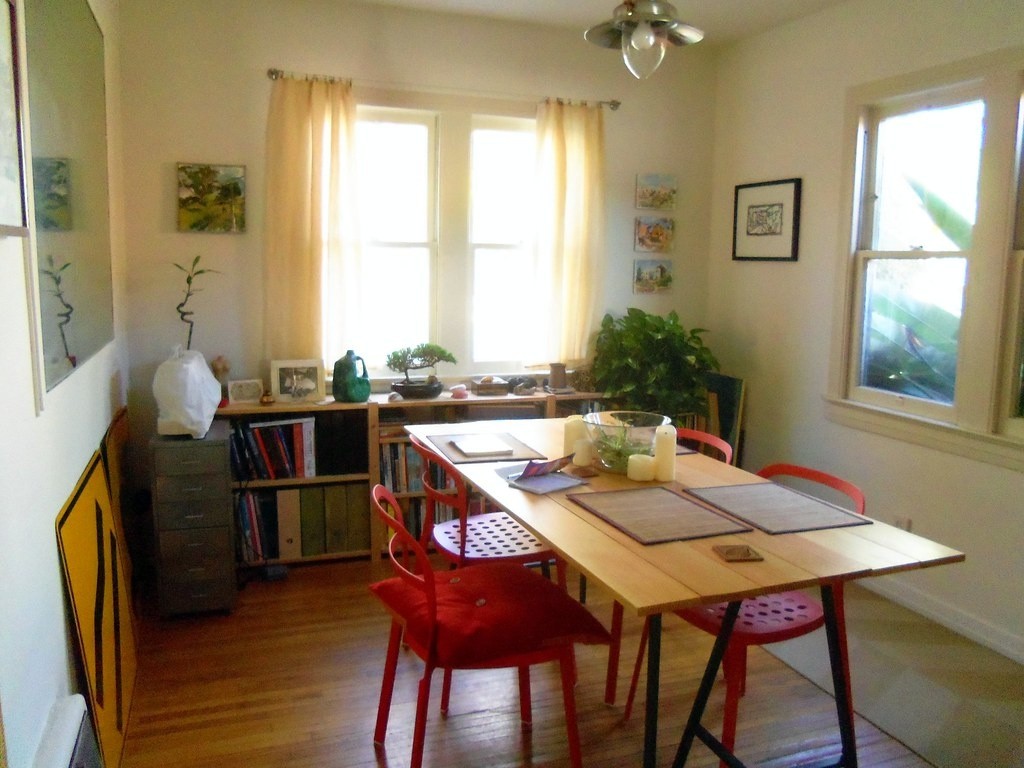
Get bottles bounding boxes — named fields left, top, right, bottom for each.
left=331, top=350, right=372, bottom=403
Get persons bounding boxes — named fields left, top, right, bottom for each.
left=285, top=373, right=316, bottom=397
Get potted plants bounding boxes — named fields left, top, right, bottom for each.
left=386, top=344, right=457, bottom=396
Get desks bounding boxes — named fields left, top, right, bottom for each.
left=401, top=416, right=964, bottom=767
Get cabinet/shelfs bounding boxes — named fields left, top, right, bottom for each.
left=218, top=382, right=630, bottom=569
left=149, top=419, right=234, bottom=618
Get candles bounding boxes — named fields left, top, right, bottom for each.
left=563, top=414, right=582, bottom=457
left=654, top=426, right=677, bottom=481
left=572, top=438, right=593, bottom=467
left=628, top=454, right=656, bottom=482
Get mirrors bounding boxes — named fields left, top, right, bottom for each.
left=15, top=1, right=123, bottom=413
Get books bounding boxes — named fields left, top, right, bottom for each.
left=230, top=418, right=512, bottom=558
left=509, top=453, right=591, bottom=495
left=545, top=384, right=576, bottom=394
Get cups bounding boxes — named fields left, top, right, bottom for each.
left=549, top=363, right=566, bottom=388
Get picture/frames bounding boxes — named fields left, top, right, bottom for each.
left=731, top=177, right=802, bottom=262
left=269, top=359, right=324, bottom=403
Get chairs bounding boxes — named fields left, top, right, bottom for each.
left=370, top=485, right=584, bottom=767
left=406, top=433, right=569, bottom=592
left=598, top=426, right=733, bottom=705
left=627, top=463, right=866, bottom=767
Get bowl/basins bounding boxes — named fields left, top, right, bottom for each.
left=581, top=410, right=673, bottom=476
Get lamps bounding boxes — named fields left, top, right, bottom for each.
left=585, top=0, right=705, bottom=79
